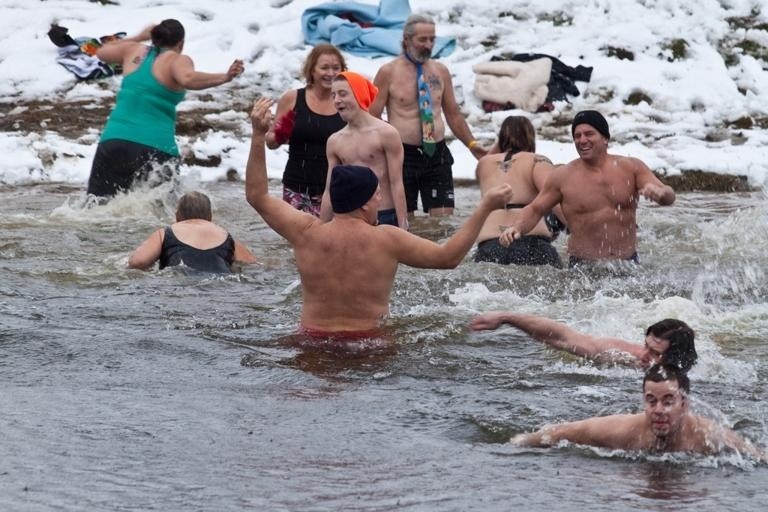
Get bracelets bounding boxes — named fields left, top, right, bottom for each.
left=467, top=141, right=479, bottom=150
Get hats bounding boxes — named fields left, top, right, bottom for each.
left=334, top=71, right=380, bottom=112
left=571, top=110, right=611, bottom=141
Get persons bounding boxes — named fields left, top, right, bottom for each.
left=129, top=191, right=253, bottom=282
left=504, top=371, right=767, bottom=465
left=264, top=45, right=355, bottom=215
left=502, top=112, right=675, bottom=269
left=366, top=14, right=489, bottom=221
left=79, top=19, right=243, bottom=209
left=316, top=73, right=411, bottom=235
left=475, top=114, right=569, bottom=267
left=246, top=95, right=512, bottom=358
left=468, top=307, right=704, bottom=375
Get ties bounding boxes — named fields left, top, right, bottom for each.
left=417, top=62, right=437, bottom=158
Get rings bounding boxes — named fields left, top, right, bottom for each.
left=252, top=107, right=257, bottom=110
